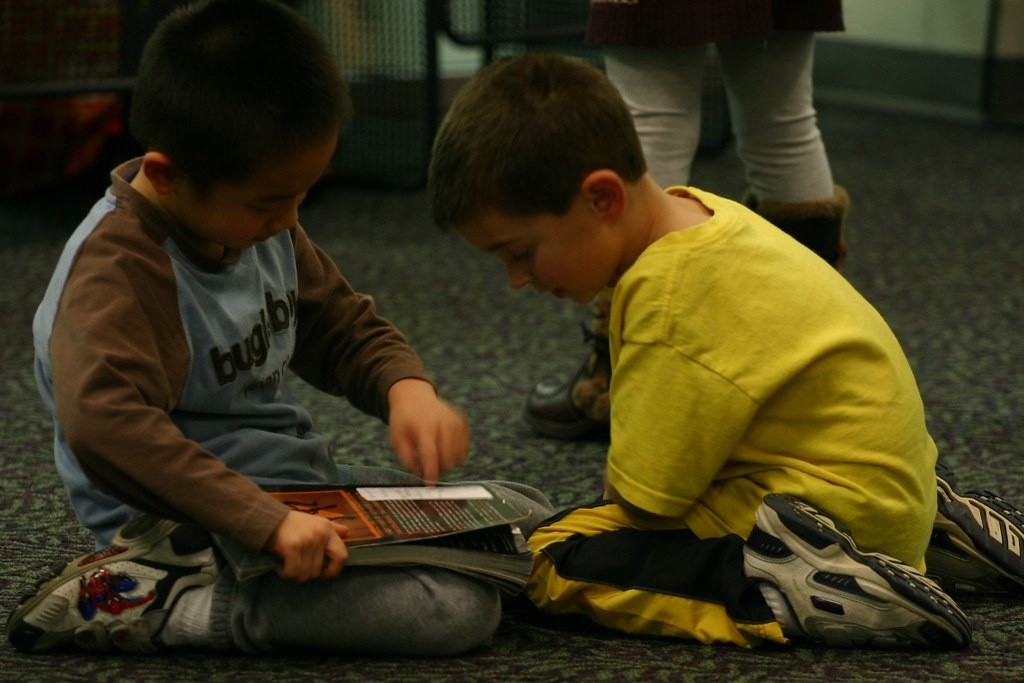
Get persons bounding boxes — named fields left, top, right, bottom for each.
left=426, top=50, right=1023, bottom=645
left=524, top=0, right=848, bottom=440
left=3, top=1, right=558, bottom=661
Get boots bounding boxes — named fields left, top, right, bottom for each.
left=523, top=277, right=617, bottom=435
left=748, top=189, right=849, bottom=270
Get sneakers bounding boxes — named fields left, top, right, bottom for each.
left=8, top=507, right=217, bottom=659
left=925, top=459, right=1024, bottom=599
left=744, top=494, right=974, bottom=647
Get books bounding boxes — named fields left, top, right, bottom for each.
left=207, top=481, right=537, bottom=596
